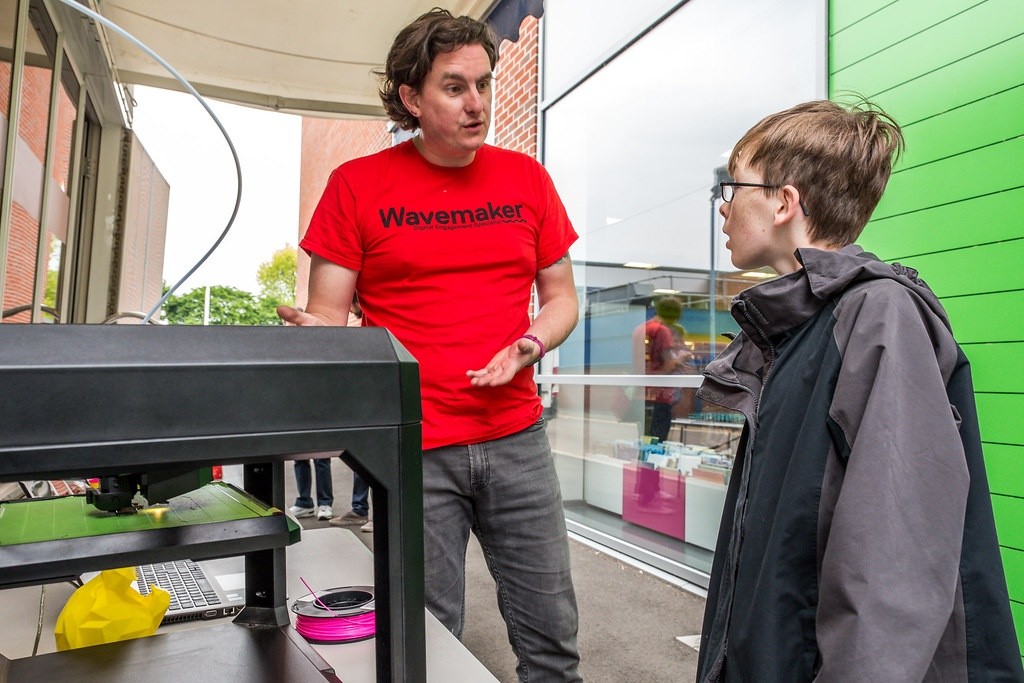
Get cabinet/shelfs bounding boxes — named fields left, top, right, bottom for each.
left=0, top=321, right=427, bottom=683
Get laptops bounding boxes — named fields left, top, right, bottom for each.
left=19, top=480, right=247, bottom=629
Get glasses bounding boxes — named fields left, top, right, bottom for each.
left=719, top=180, right=810, bottom=216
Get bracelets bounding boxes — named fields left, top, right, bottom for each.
left=513, top=334, right=546, bottom=368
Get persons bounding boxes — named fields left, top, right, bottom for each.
left=290, top=457, right=374, bottom=531
left=275, top=6, right=579, bottom=683
left=693, top=98, right=1024, bottom=683
left=629, top=295, right=695, bottom=513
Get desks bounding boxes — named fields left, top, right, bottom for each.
left=0, top=525, right=506, bottom=683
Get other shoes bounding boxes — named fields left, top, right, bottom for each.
left=317, top=505, right=333, bottom=519
left=328, top=511, right=368, bottom=525
left=290, top=505, right=315, bottom=518
left=361, top=520, right=373, bottom=531
